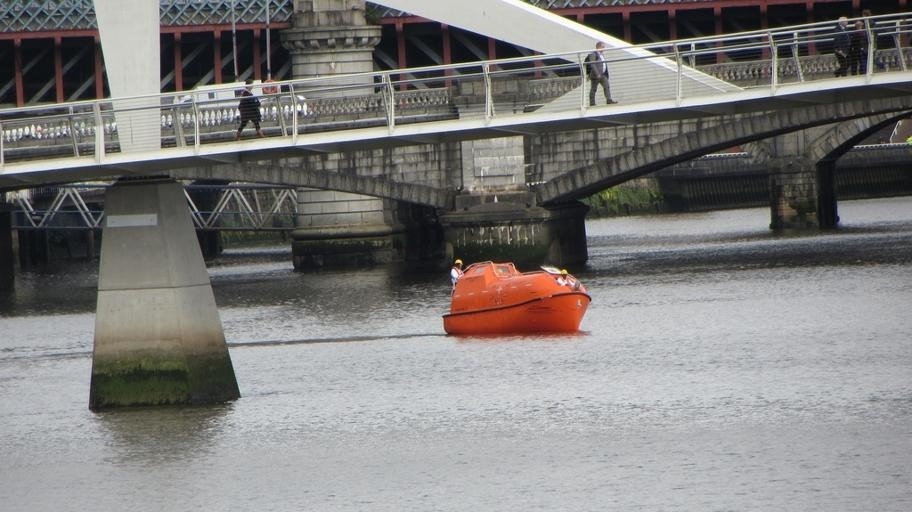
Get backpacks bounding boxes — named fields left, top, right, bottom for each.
left=585, top=51, right=599, bottom=74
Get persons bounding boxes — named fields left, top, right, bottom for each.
left=448, top=258, right=465, bottom=296
left=831, top=16, right=850, bottom=78
left=861, top=10, right=891, bottom=75
left=582, top=41, right=619, bottom=108
left=557, top=269, right=581, bottom=289
left=849, top=21, right=868, bottom=75
left=233, top=77, right=266, bottom=143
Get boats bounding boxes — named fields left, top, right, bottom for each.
left=440, top=258, right=593, bottom=335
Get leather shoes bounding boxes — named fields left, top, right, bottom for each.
left=607, top=100, right=617, bottom=104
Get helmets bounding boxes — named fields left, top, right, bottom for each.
left=561, top=269, right=568, bottom=275
left=455, top=259, right=463, bottom=264
